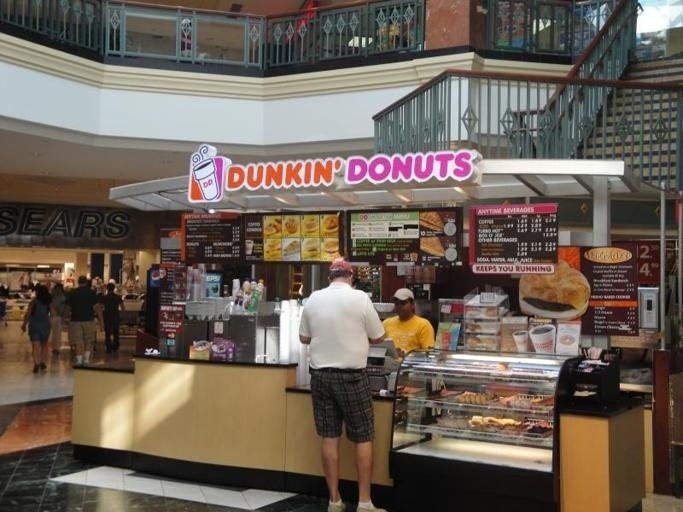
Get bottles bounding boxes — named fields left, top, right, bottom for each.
left=231, top=277, right=266, bottom=312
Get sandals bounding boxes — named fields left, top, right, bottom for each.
left=145, top=348, right=160, bottom=355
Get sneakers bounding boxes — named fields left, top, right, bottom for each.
left=357, top=506, right=387, bottom=512
left=33, top=363, right=39, bottom=374
left=40, top=362, right=47, bottom=370
left=327, top=502, right=347, bottom=512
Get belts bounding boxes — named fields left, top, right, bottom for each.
left=318, top=369, right=362, bottom=373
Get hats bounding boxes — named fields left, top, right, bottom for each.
left=393, top=287, right=414, bottom=301
left=329, top=261, right=354, bottom=273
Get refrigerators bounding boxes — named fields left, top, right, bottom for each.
left=146, top=263, right=159, bottom=335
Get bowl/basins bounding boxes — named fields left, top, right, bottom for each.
left=372, top=302, right=395, bottom=313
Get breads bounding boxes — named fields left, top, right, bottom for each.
left=469, top=416, right=522, bottom=429
left=285, top=218, right=299, bottom=234
left=455, top=390, right=489, bottom=408
left=518, top=257, right=591, bottom=317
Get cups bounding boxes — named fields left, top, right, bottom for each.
left=279, top=298, right=311, bottom=387
left=529, top=323, right=557, bottom=353
left=173, top=271, right=185, bottom=290
left=244, top=239, right=253, bottom=256
left=186, top=262, right=206, bottom=304
left=159, top=267, right=165, bottom=278
left=512, top=331, right=530, bottom=354
left=441, top=328, right=451, bottom=350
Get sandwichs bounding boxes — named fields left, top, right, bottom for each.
left=283, top=239, right=300, bottom=259
left=420, top=212, right=446, bottom=257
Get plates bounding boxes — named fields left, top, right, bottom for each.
left=211, top=344, right=225, bottom=353
left=444, top=223, right=457, bottom=236
left=193, top=340, right=210, bottom=351
left=445, top=247, right=458, bottom=262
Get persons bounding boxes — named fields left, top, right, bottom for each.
left=380, top=288, right=435, bottom=363
left=0, top=276, right=125, bottom=374
left=299, top=259, right=386, bottom=511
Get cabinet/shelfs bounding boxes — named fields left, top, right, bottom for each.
left=390, top=347, right=583, bottom=512
left=462, top=290, right=509, bottom=353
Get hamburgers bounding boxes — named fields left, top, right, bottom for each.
left=323, top=240, right=340, bottom=258
left=304, top=241, right=320, bottom=258
left=322, top=216, right=339, bottom=234
